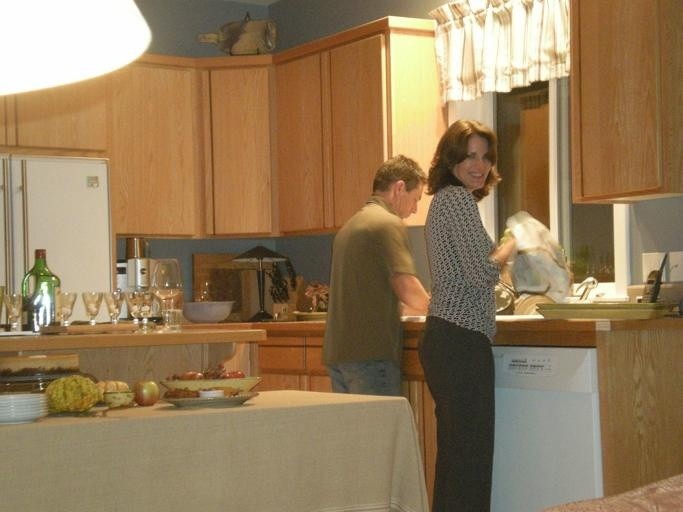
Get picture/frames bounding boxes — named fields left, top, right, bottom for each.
left=191, top=253, right=251, bottom=324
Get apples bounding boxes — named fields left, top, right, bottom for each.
left=132, top=380, right=160, bottom=406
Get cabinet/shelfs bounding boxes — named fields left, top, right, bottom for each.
left=272, top=16, right=444, bottom=235
left=3, top=55, right=200, bottom=242
left=201, top=50, right=274, bottom=235
left=399, top=327, right=441, bottom=512
left=254, top=330, right=336, bottom=510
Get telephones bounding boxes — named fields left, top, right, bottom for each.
left=641, top=253, right=668, bottom=303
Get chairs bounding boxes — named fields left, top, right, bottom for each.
left=569, top=0, right=682, bottom=204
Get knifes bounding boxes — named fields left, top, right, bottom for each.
left=266, top=260, right=296, bottom=303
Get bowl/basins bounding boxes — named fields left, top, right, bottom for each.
left=103, top=391, right=136, bottom=408
left=182, top=300, right=235, bottom=323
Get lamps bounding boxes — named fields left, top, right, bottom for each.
left=229, top=244, right=290, bottom=322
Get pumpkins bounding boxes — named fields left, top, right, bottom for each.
left=46, top=375, right=98, bottom=413
left=97, top=380, right=129, bottom=403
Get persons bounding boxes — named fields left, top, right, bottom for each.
left=321, top=154, right=430, bottom=395
left=418, top=119, right=517, bottom=512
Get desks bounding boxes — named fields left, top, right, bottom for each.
left=0, top=329, right=268, bottom=401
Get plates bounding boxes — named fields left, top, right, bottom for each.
left=292, top=311, right=327, bottom=321
left=493, top=286, right=556, bottom=316
left=0, top=394, right=50, bottom=424
left=159, top=377, right=262, bottom=410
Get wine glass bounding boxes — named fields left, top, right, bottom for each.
left=81, top=292, right=103, bottom=325
left=151, top=258, right=182, bottom=331
left=126, top=288, right=153, bottom=333
left=60, top=293, right=76, bottom=326
left=2, top=295, right=21, bottom=332
left=104, top=293, right=125, bottom=324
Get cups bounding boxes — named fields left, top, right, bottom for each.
left=123, top=237, right=151, bottom=259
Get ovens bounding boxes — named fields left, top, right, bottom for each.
left=490, top=347, right=603, bottom=512
